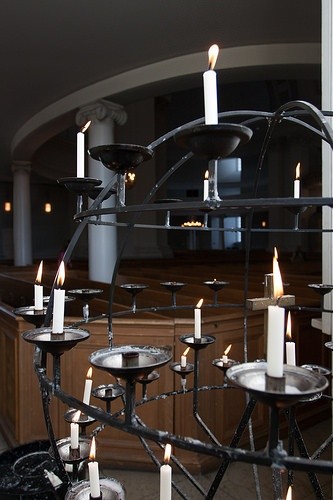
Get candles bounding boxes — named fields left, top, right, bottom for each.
left=76, top=120, right=91, bottom=177
left=203, top=43, right=219, bottom=125
left=285, top=310, right=296, bottom=366
left=87, top=436, right=101, bottom=498
left=160, top=443, right=172, bottom=500
left=70, top=410, right=81, bottom=449
left=82, top=367, right=93, bottom=405
left=267, top=247, right=285, bottom=378
left=193, top=298, right=204, bottom=340
left=34, top=260, right=45, bottom=310
left=203, top=170, right=209, bottom=201
left=180, top=347, right=190, bottom=367
left=294, top=163, right=300, bottom=198
left=52, top=260, right=66, bottom=334
left=222, top=344, right=232, bottom=364
